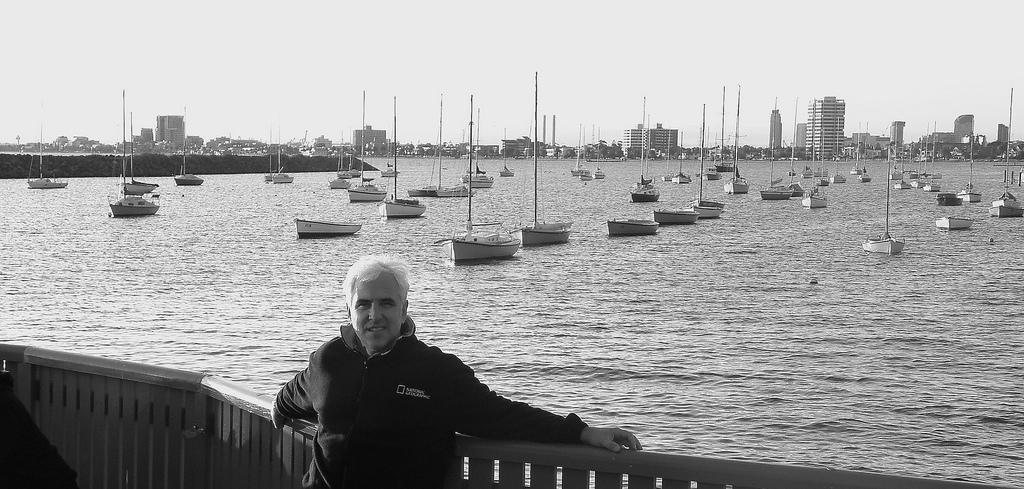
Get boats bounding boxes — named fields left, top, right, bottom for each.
left=935, top=216, right=974, bottom=231
left=606, top=216, right=661, bottom=238
left=652, top=208, right=700, bottom=225
left=934, top=192, right=964, bottom=207
left=292, top=218, right=363, bottom=237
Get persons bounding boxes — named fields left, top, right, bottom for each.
left=271, top=251, right=646, bottom=488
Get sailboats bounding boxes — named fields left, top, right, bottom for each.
left=886, top=120, right=944, bottom=193
left=569, top=121, right=594, bottom=182
left=758, top=96, right=792, bottom=201
left=694, top=82, right=738, bottom=181
left=629, top=97, right=662, bottom=203
left=828, top=117, right=847, bottom=183
left=174, top=139, right=204, bottom=185
left=262, top=123, right=294, bottom=184
left=380, top=138, right=399, bottom=178
left=988, top=86, right=1024, bottom=219
left=690, top=104, right=727, bottom=219
left=660, top=129, right=675, bottom=182
left=344, top=90, right=389, bottom=203
left=782, top=96, right=833, bottom=207
left=326, top=132, right=361, bottom=189
left=27, top=123, right=68, bottom=188
left=110, top=89, right=159, bottom=215
left=955, top=135, right=983, bottom=203
left=433, top=94, right=521, bottom=264
left=858, top=147, right=906, bottom=255
left=506, top=70, right=574, bottom=247
left=405, top=131, right=438, bottom=197
left=857, top=139, right=872, bottom=183
left=723, top=84, right=750, bottom=195
left=500, top=127, right=515, bottom=177
left=848, top=130, right=862, bottom=175
left=591, top=127, right=606, bottom=179
left=670, top=132, right=693, bottom=184
left=434, top=93, right=478, bottom=198
left=459, top=108, right=495, bottom=189
left=377, top=96, right=427, bottom=218
